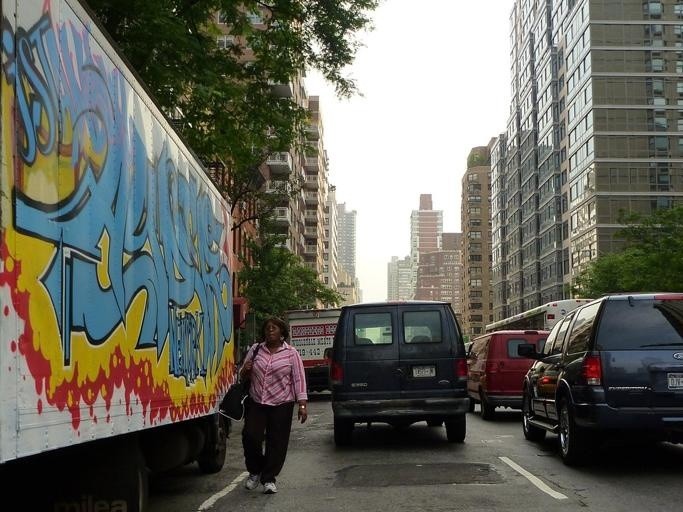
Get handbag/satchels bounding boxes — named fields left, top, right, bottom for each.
left=219, top=382, right=249, bottom=422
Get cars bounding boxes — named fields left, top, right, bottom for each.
left=518, top=294, right=683, bottom=463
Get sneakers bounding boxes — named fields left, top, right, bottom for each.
left=245, top=473, right=259, bottom=490
left=262, top=481, right=277, bottom=493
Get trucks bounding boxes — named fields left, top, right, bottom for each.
left=283, top=309, right=342, bottom=400
left=0, top=2, right=239, bottom=512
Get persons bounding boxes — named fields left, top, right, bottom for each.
left=239, top=318, right=308, bottom=494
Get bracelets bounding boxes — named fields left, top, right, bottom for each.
left=299, top=405, right=306, bottom=409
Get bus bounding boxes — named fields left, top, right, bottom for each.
left=486, top=299, right=596, bottom=334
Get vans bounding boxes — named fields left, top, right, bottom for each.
left=324, top=300, right=470, bottom=445
left=468, top=330, right=550, bottom=416
